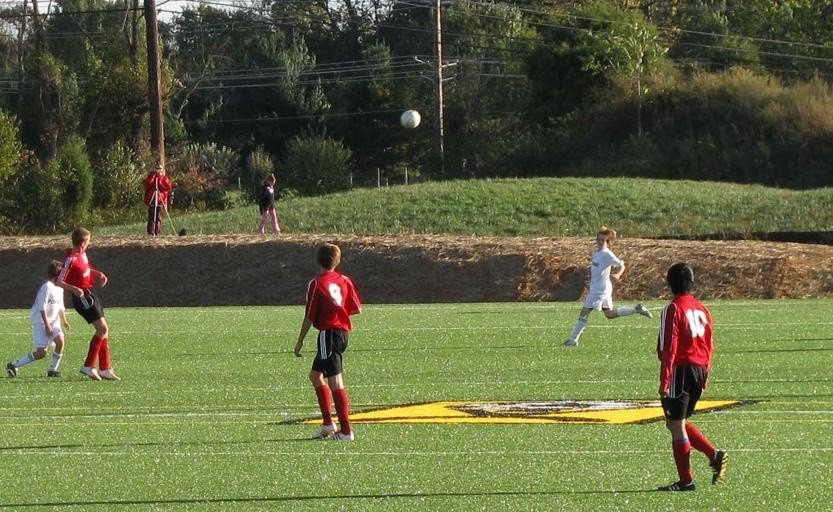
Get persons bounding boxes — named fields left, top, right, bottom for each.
left=657, top=261, right=729, bottom=491
left=295, top=243, right=362, bottom=441
left=259, top=175, right=281, bottom=235
left=559, top=225, right=653, bottom=347
left=144, top=165, right=172, bottom=236
left=54, top=226, right=121, bottom=381
left=5, top=259, right=71, bottom=377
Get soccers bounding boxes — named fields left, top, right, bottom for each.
left=400, top=109, right=421, bottom=128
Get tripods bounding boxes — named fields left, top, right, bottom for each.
left=138, top=189, right=180, bottom=237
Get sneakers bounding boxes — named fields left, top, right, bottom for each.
left=708, top=450, right=729, bottom=486
left=636, top=303, right=653, bottom=319
left=79, top=364, right=101, bottom=381
left=327, top=431, right=354, bottom=442
left=559, top=339, right=577, bottom=347
left=312, top=422, right=338, bottom=440
left=658, top=480, right=695, bottom=492
left=97, top=367, right=121, bottom=381
left=47, top=370, right=62, bottom=378
left=6, top=362, right=17, bottom=378
